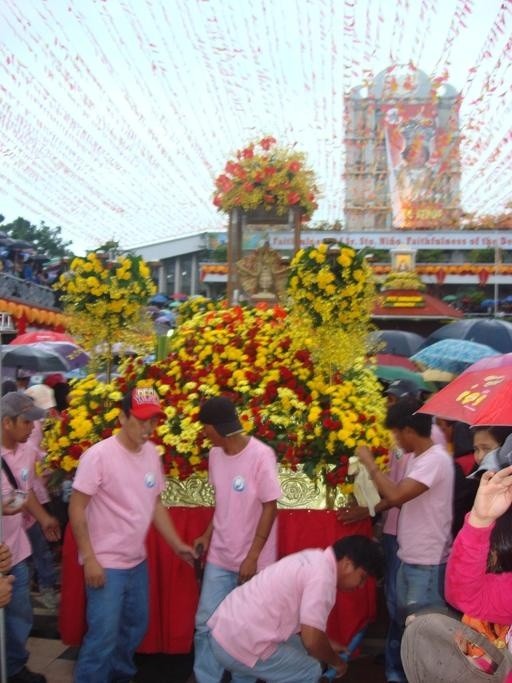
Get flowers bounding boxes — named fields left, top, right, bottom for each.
left=35, top=136, right=398, bottom=510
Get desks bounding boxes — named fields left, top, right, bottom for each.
left=61, top=462, right=377, bottom=660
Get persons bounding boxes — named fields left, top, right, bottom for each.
left=1, top=250, right=56, bottom=284
left=0, top=367, right=77, bottom=682
left=205, top=534, right=383, bottom=683
left=335, top=378, right=511, bottom=683
left=190, top=395, right=284, bottom=683
left=67, top=384, right=203, bottom=683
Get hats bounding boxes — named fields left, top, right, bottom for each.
left=17, top=367, right=34, bottom=379
left=122, top=387, right=168, bottom=421
left=384, top=378, right=418, bottom=400
left=200, top=397, right=245, bottom=436
left=26, top=384, right=57, bottom=410
left=0, top=391, right=46, bottom=421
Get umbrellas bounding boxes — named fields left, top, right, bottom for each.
left=0, top=212, right=71, bottom=270
left=443, top=294, right=512, bottom=309
left=0, top=295, right=204, bottom=383
left=365, top=317, right=512, bottom=430
